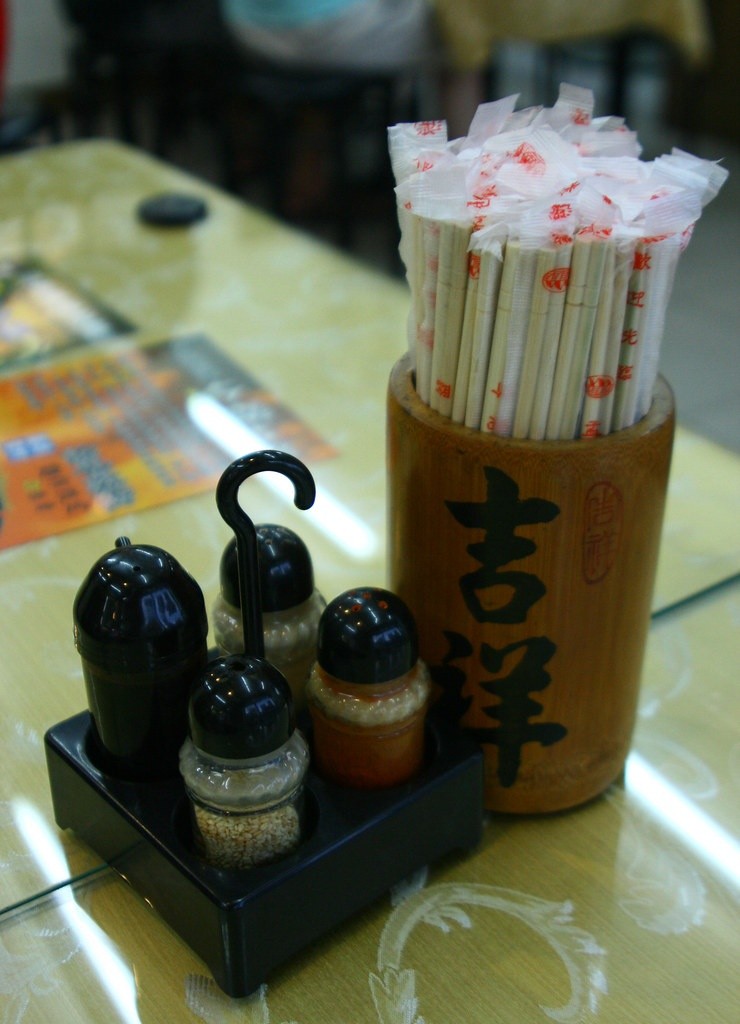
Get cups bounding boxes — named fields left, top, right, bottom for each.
left=384, top=351, right=676, bottom=816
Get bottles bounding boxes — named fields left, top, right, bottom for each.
left=178, top=653, right=311, bottom=867
left=210, top=524, right=327, bottom=708
left=71, top=536, right=208, bottom=764
left=308, top=585, right=428, bottom=786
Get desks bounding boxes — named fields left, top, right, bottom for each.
left=0, top=139, right=740, bottom=1024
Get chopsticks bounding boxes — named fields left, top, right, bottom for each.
left=395, top=188, right=679, bottom=439
